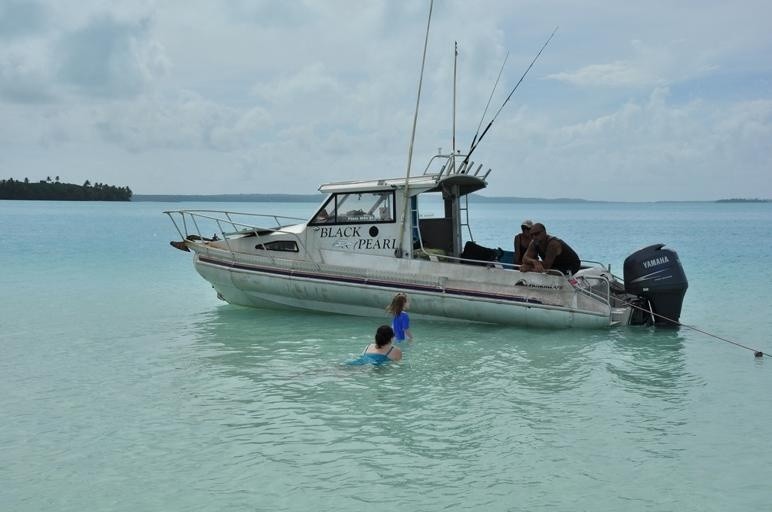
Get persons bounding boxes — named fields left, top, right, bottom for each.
left=335, top=324, right=402, bottom=371
left=512, top=220, right=540, bottom=270
left=519, top=223, right=581, bottom=278
left=383, top=290, right=413, bottom=342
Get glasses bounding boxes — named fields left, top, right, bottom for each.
left=531, top=231, right=540, bottom=236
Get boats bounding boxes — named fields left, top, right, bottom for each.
left=162, top=144, right=690, bottom=334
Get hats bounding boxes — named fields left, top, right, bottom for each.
left=520, top=220, right=533, bottom=228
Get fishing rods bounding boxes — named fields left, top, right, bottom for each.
left=398, top=1, right=434, bottom=257
left=590, top=285, right=771, bottom=358
left=456, top=24, right=558, bottom=175
